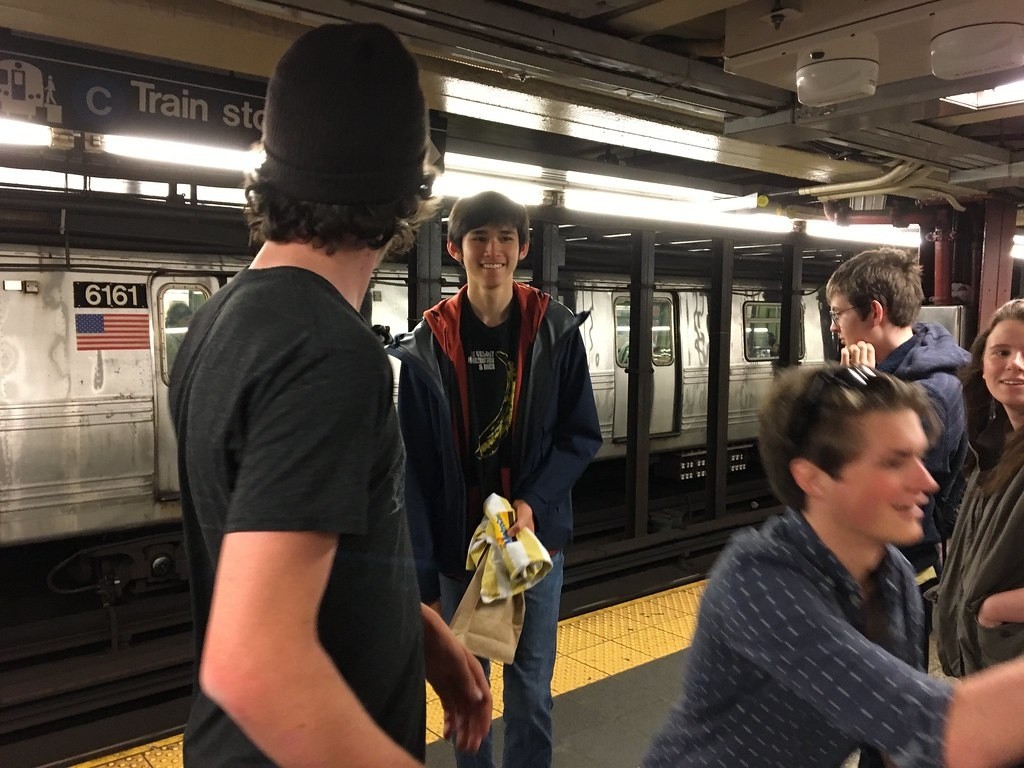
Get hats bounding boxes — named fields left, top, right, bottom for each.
left=261, top=22, right=429, bottom=205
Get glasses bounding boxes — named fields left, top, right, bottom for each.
left=827, top=305, right=855, bottom=322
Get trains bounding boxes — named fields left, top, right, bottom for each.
left=0, top=244, right=850, bottom=625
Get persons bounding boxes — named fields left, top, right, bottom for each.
left=397, top=193, right=604, bottom=767
left=166, top=23, right=1024, bottom=768
